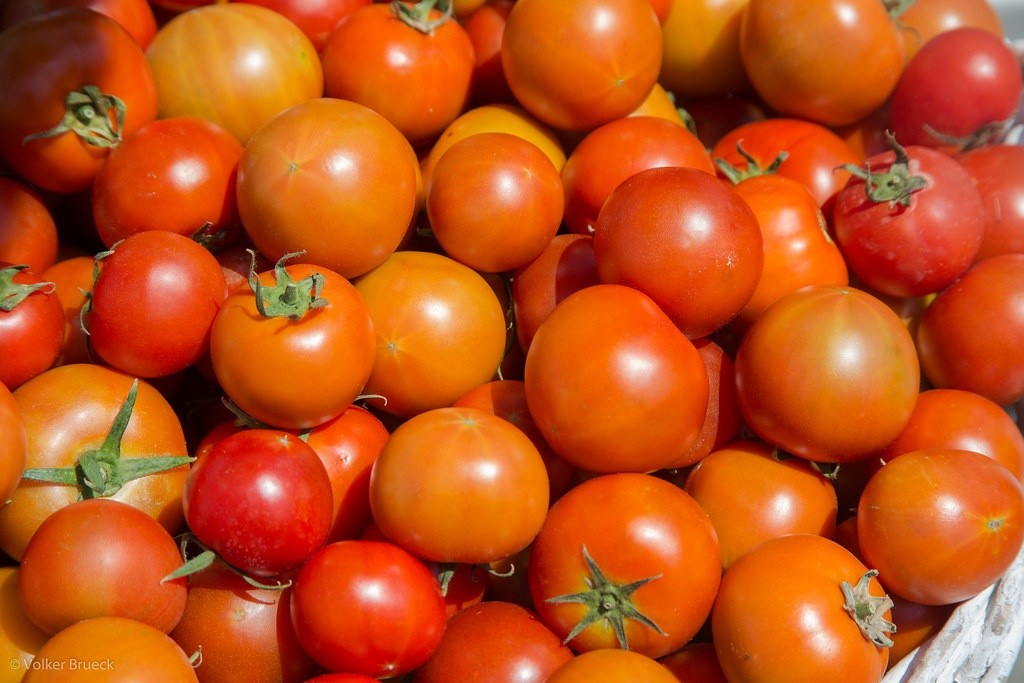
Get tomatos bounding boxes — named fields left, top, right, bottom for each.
left=0, top=0, right=1023, bottom=683
